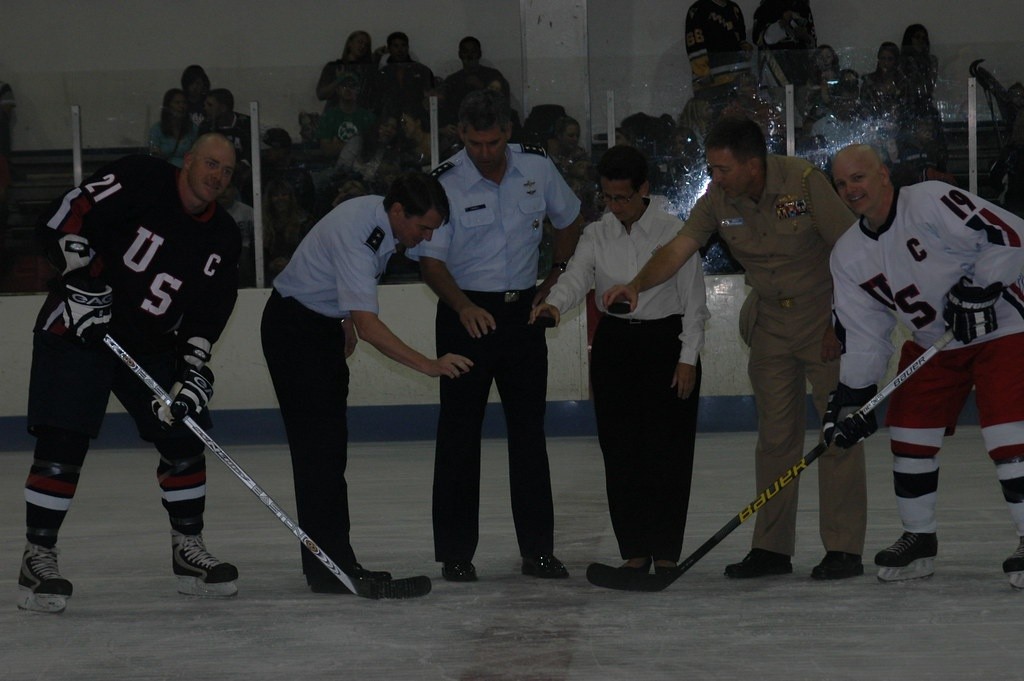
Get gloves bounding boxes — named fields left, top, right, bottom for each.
left=64, top=281, right=115, bottom=354
left=942, top=275, right=1002, bottom=344
left=150, top=363, right=215, bottom=428
left=823, top=381, right=879, bottom=448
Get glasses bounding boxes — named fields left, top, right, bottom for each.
left=598, top=186, right=639, bottom=204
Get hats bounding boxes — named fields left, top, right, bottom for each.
left=334, top=71, right=359, bottom=86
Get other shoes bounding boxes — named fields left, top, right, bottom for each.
left=622, top=555, right=650, bottom=571
left=652, top=559, right=676, bottom=569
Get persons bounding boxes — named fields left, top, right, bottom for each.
left=18, top=131, right=245, bottom=614
left=821, top=142, right=1024, bottom=591
left=407, top=93, right=584, bottom=581
left=601, top=119, right=870, bottom=581
left=527, top=145, right=712, bottom=578
left=259, top=172, right=474, bottom=595
left=0, top=0, right=1024, bottom=295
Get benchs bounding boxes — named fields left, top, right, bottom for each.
left=937, top=121, right=1009, bottom=199
left=9, top=151, right=131, bottom=278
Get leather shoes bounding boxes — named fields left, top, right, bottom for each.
left=807, top=550, right=863, bottom=580
left=336, top=564, right=392, bottom=584
left=522, top=554, right=568, bottom=578
left=441, top=553, right=476, bottom=581
left=725, top=549, right=793, bottom=579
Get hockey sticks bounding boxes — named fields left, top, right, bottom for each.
left=103, top=334, right=432, bottom=601
left=586, top=328, right=954, bottom=593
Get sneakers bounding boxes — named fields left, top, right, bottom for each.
left=171, top=528, right=239, bottom=595
left=874, top=531, right=938, bottom=582
left=15, top=541, right=73, bottom=612
left=1002, top=535, right=1024, bottom=590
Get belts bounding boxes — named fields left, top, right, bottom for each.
left=760, top=282, right=833, bottom=310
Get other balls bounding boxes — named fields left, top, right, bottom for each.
left=459, top=366, right=471, bottom=375
left=534, top=316, right=555, bottom=328
left=608, top=302, right=631, bottom=314
